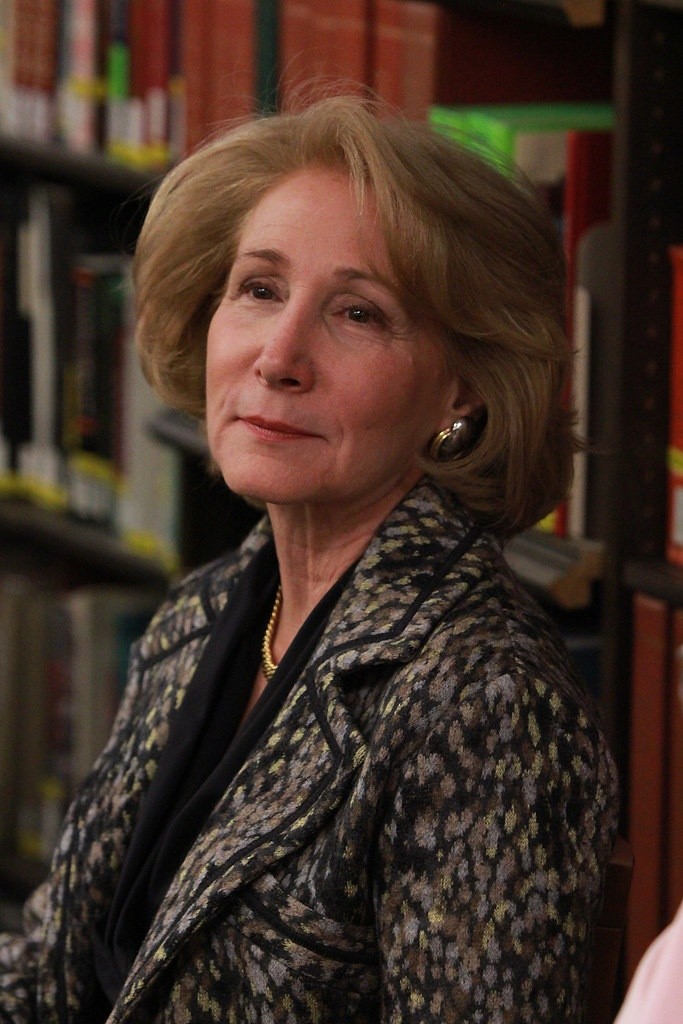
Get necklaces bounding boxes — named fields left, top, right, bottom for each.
left=260, top=586, right=280, bottom=683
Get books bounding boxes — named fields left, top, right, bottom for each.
left=0, top=543, right=168, bottom=904
left=665, top=245, right=683, bottom=567
left=0, top=0, right=615, bottom=178
left=1, top=132, right=616, bottom=573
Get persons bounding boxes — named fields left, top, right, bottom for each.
left=0, top=100, right=619, bottom=1024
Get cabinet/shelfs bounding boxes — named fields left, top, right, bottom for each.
left=0, top=0, right=683, bottom=1024
